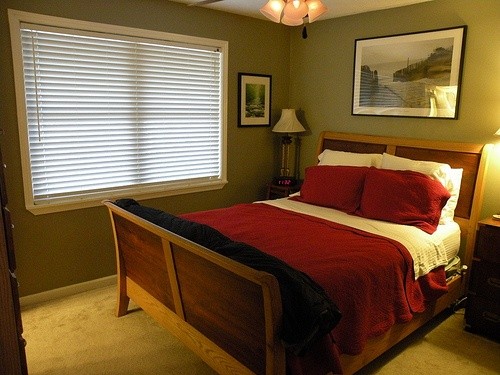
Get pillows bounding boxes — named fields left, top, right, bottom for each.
left=287, top=149, right=464, bottom=235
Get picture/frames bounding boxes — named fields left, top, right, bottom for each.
left=352, top=25, right=467, bottom=120
left=238, top=72, right=271, bottom=127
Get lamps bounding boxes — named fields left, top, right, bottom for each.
left=272, top=108, right=307, bottom=176
left=259, top=0, right=327, bottom=40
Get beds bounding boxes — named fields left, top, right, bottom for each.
left=101, top=132, right=487, bottom=375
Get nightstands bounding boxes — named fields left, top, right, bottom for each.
left=463, top=217, right=500, bottom=342
left=266, top=183, right=301, bottom=200
